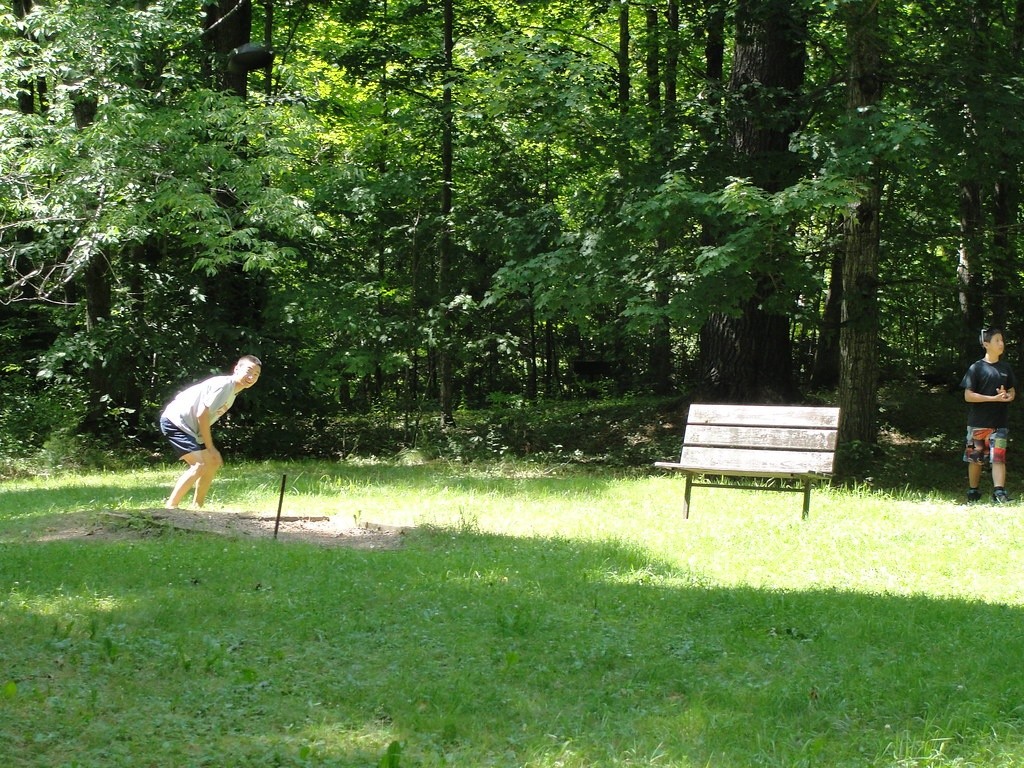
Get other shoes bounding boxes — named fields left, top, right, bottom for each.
left=966, top=488, right=980, bottom=504
left=993, top=487, right=1011, bottom=502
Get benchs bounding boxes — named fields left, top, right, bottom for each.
left=651, top=403, right=844, bottom=519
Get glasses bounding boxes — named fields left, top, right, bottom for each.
left=981, top=328, right=989, bottom=344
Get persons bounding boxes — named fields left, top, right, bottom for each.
left=160, top=355, right=262, bottom=508
left=959, top=329, right=1015, bottom=503
